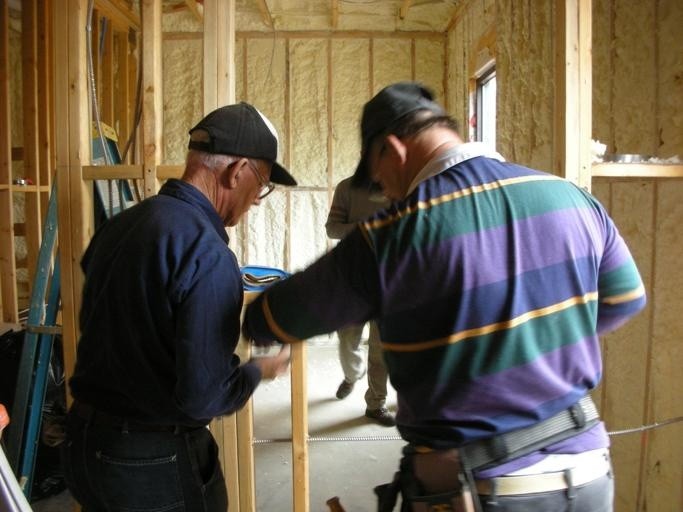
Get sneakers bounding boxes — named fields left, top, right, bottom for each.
left=366, top=407, right=396, bottom=427
left=336, top=367, right=366, bottom=399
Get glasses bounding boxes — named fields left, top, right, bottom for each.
left=368, top=143, right=392, bottom=204
left=246, top=157, right=276, bottom=200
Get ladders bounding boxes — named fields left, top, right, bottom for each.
left=5, top=120, right=141, bottom=502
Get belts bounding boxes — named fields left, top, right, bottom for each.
left=402, top=394, right=598, bottom=494
left=67, top=400, right=202, bottom=432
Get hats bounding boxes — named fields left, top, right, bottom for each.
left=350, top=82, right=442, bottom=192
left=190, top=101, right=298, bottom=187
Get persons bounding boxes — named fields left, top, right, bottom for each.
left=239, top=79, right=649, bottom=512
left=58, top=100, right=298, bottom=512
left=324, top=142, right=398, bottom=427
